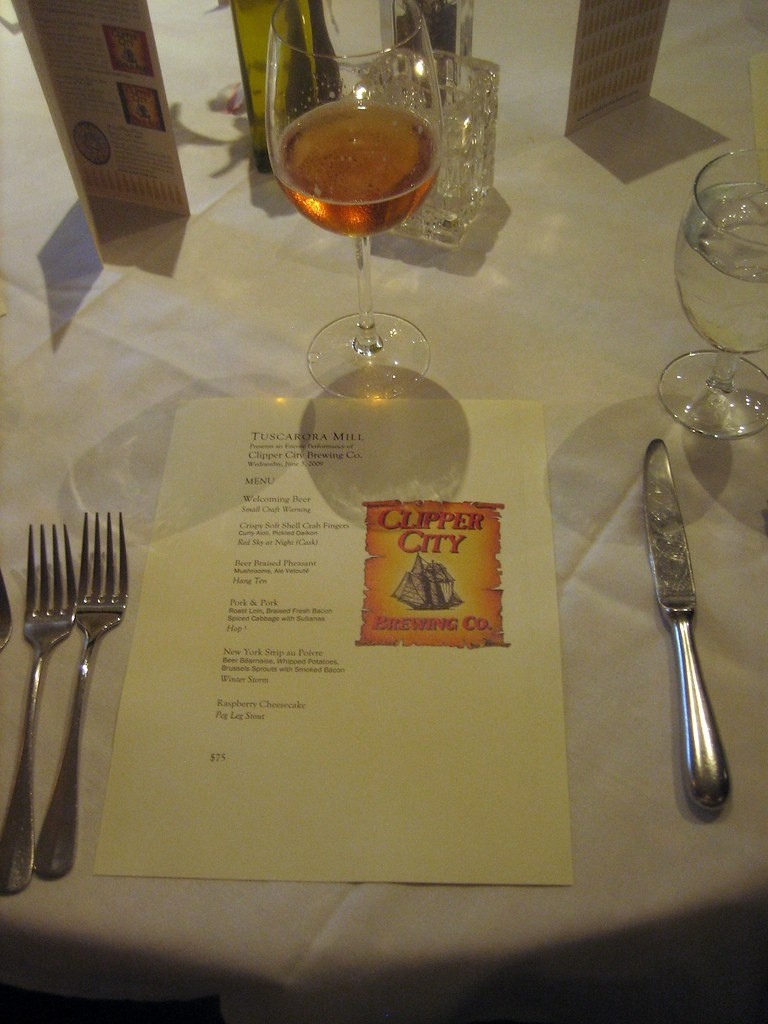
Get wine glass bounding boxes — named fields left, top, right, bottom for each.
left=266, top=1, right=447, bottom=402
left=659, top=149, right=768, bottom=440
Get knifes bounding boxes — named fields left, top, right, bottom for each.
left=644, top=439, right=729, bottom=813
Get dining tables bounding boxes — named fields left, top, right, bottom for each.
left=0, top=0, right=768, bottom=1019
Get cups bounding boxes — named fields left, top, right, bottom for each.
left=229, top=0, right=342, bottom=174
left=352, top=47, right=500, bottom=246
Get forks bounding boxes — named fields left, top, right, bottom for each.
left=34, top=511, right=129, bottom=879
left=1, top=524, right=76, bottom=895
